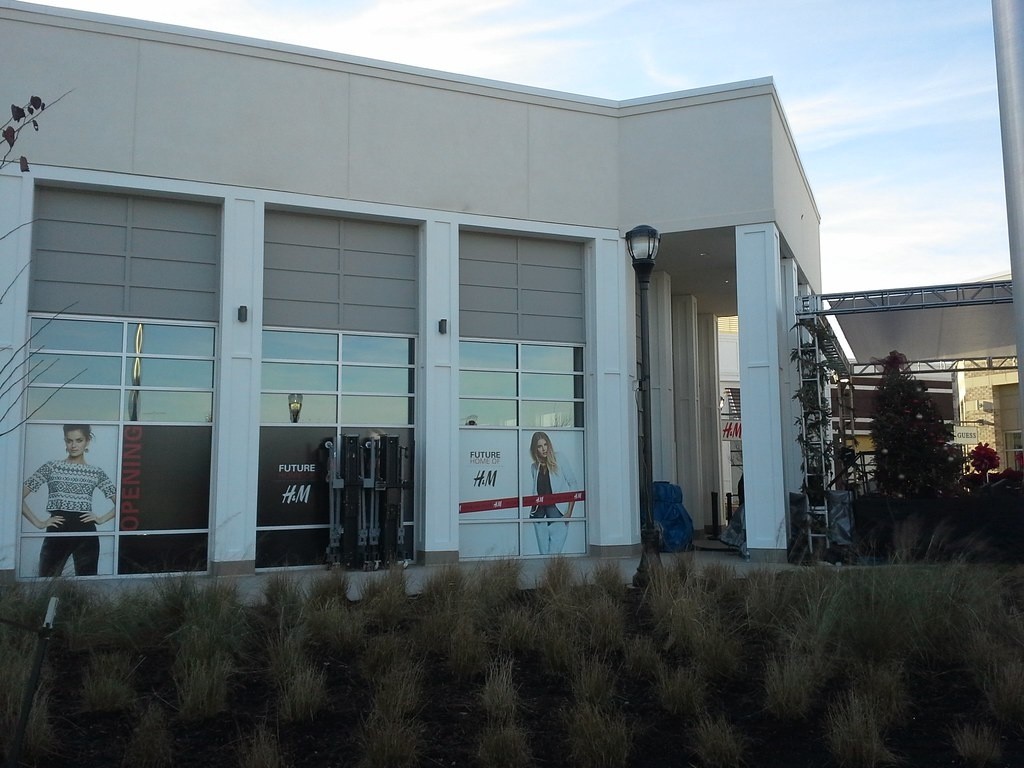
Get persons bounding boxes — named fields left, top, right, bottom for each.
left=22, top=424, right=117, bottom=577
left=528, top=432, right=575, bottom=555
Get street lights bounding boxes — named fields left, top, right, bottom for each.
left=625, top=223, right=674, bottom=590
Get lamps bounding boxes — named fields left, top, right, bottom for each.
left=288, top=393, right=303, bottom=423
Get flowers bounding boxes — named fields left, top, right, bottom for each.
left=968, top=442, right=1001, bottom=473
